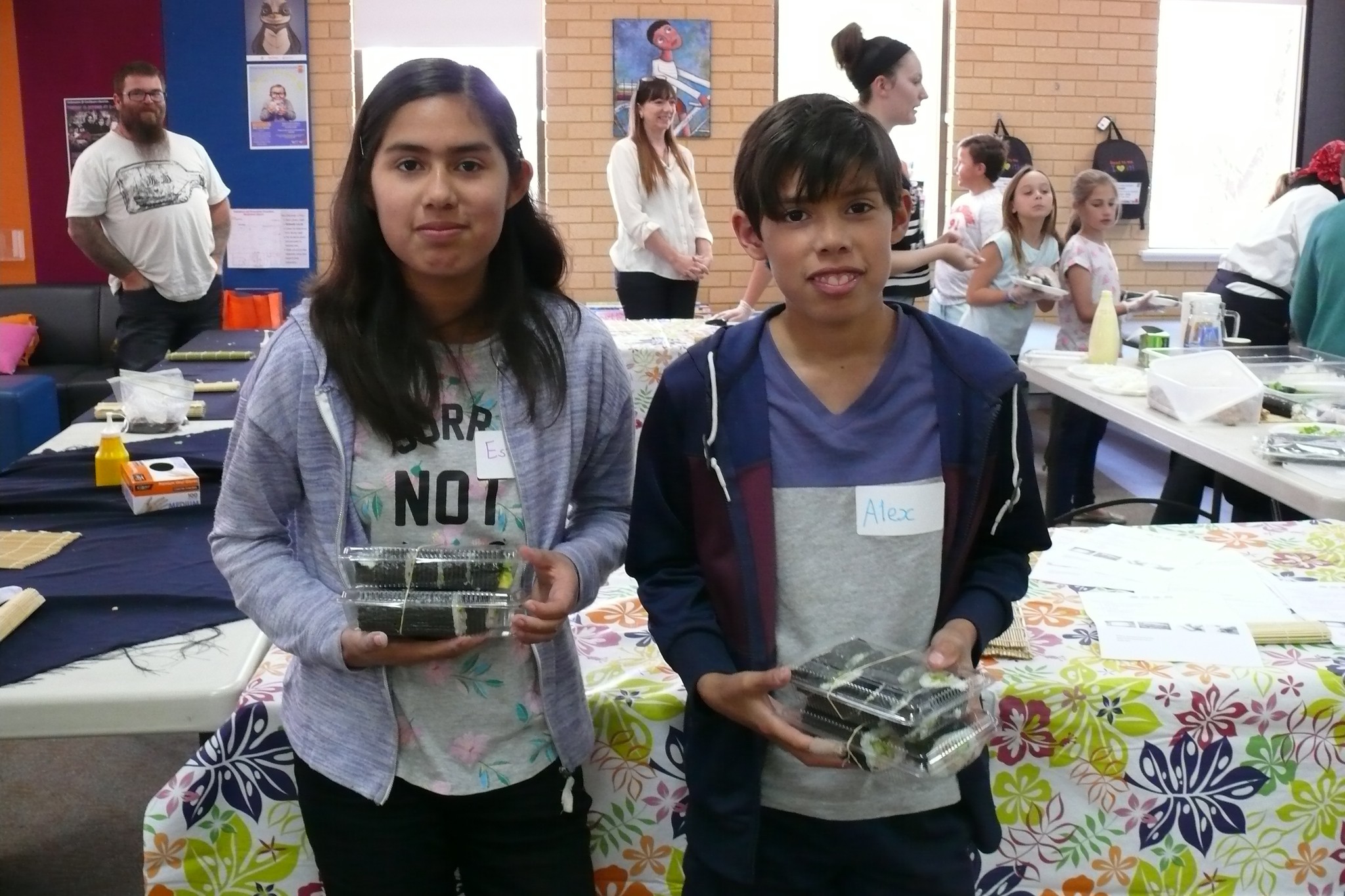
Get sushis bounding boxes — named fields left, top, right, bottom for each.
left=355, top=548, right=511, bottom=640
left=1261, top=391, right=1303, bottom=419
left=791, top=640, right=979, bottom=776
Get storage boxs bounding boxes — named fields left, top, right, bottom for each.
left=120, top=457, right=202, bottom=515
left=1140, top=342, right=1345, bottom=429
left=605, top=319, right=745, bottom=448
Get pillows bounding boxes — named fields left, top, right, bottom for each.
left=0, top=314, right=39, bottom=373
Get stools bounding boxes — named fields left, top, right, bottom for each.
left=0, top=374, right=59, bottom=471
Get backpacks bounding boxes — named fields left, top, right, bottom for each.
left=995, top=119, right=1032, bottom=178
left=1092, top=121, right=1149, bottom=219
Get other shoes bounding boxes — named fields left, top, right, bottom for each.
left=1074, top=507, right=1127, bottom=524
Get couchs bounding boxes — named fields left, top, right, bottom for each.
left=0, top=284, right=121, bottom=428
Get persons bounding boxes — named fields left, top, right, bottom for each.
left=833, top=23, right=987, bottom=308
left=66, top=60, right=233, bottom=377
left=260, top=85, right=296, bottom=122
left=714, top=259, right=773, bottom=323
left=68, top=111, right=119, bottom=152
left=1150, top=140, right=1345, bottom=525
left=607, top=76, right=714, bottom=319
left=1043, top=169, right=1166, bottom=528
left=626, top=94, right=1052, bottom=896
left=210, top=59, right=638, bottom=896
left=929, top=135, right=1003, bottom=325
left=958, top=165, right=1065, bottom=367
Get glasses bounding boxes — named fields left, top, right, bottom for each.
left=637, top=76, right=667, bottom=92
left=116, top=89, right=168, bottom=102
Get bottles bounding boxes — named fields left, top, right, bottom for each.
left=94, top=412, right=135, bottom=486
left=1087, top=290, right=1128, bottom=364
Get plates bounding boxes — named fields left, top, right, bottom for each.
left=1126, top=296, right=1182, bottom=307
left=1068, top=363, right=1148, bottom=396
left=1010, top=276, right=1069, bottom=296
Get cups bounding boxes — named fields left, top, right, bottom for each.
left=1181, top=300, right=1241, bottom=350
left=1221, top=337, right=1251, bottom=347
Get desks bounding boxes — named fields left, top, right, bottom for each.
left=143, top=519, right=1345, bottom=896
left=0, top=329, right=329, bottom=746
left=1020, top=352, right=1345, bottom=521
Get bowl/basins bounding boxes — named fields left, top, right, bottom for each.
left=1145, top=350, right=1268, bottom=429
left=774, top=630, right=1002, bottom=780
left=335, top=544, right=527, bottom=643
